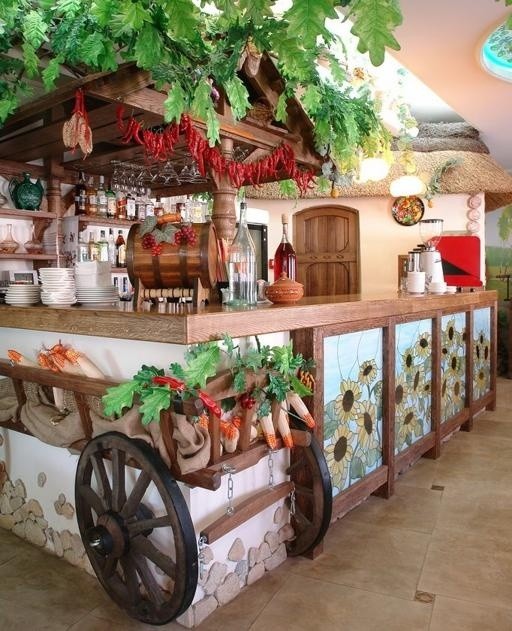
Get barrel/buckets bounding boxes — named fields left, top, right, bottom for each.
left=123, top=222, right=222, bottom=290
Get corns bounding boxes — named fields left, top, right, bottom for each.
left=198, top=390, right=316, bottom=454
left=7, top=347, right=116, bottom=422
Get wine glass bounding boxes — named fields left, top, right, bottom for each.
left=110, top=151, right=212, bottom=193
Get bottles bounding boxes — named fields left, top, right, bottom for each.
left=75, top=169, right=195, bottom=267
left=227, top=200, right=259, bottom=307
left=0, top=220, right=44, bottom=254
left=264, top=272, right=305, bottom=307
left=7, top=171, right=45, bottom=211
left=257, top=279, right=266, bottom=302
left=274, top=212, right=296, bottom=282
left=114, top=275, right=130, bottom=302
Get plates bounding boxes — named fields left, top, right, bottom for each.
left=5, top=263, right=119, bottom=309
left=407, top=270, right=457, bottom=294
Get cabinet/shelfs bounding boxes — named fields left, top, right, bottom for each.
left=62, top=217, right=132, bottom=274
left=0, top=208, right=57, bottom=261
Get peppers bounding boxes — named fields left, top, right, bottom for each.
left=71, top=88, right=318, bottom=199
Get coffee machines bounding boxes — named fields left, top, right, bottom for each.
left=409, top=218, right=447, bottom=282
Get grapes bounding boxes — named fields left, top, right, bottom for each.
left=142, top=224, right=199, bottom=259
